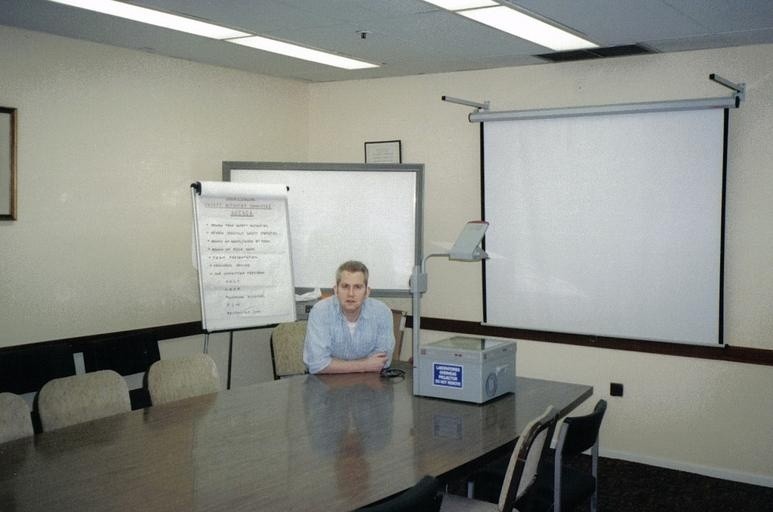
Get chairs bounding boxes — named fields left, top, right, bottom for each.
left=0, top=319, right=606, bottom=512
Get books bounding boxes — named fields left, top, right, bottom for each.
left=187, top=176, right=299, bottom=331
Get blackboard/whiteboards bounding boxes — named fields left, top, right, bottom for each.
left=223, top=161, right=425, bottom=297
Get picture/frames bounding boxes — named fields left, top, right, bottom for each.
left=0, top=106, right=17, bottom=221
left=363, top=139, right=401, bottom=164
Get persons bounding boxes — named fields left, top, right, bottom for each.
left=299, top=259, right=396, bottom=375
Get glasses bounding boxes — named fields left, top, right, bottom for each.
left=379, top=367, right=406, bottom=378
